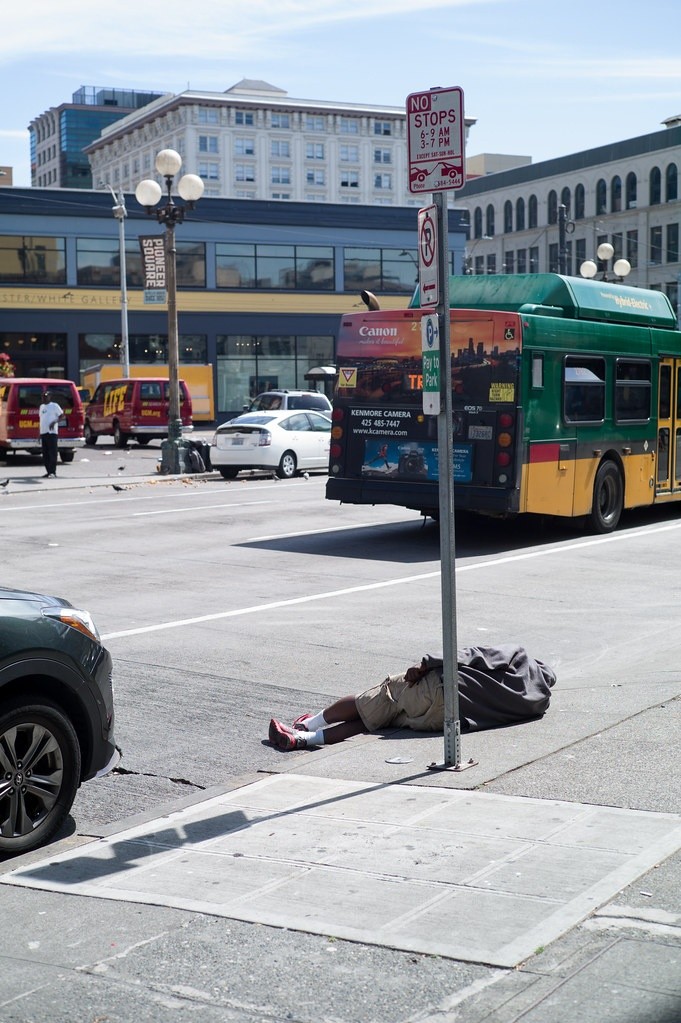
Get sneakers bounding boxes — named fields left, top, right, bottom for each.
left=292, top=715, right=312, bottom=732
left=268, top=718, right=307, bottom=749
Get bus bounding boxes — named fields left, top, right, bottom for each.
left=324, top=271, right=681, bottom=536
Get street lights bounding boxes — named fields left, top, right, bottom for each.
left=578, top=242, right=633, bottom=284
left=136, top=148, right=206, bottom=474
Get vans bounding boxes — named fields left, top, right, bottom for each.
left=0, top=377, right=93, bottom=461
left=83, top=377, right=194, bottom=448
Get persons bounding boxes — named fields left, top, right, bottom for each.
left=365, top=444, right=392, bottom=470
left=38, top=391, right=64, bottom=478
left=269, top=645, right=557, bottom=751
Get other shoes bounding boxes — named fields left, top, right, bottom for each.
left=44, top=473, right=56, bottom=478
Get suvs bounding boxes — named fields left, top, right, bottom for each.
left=242, top=388, right=333, bottom=420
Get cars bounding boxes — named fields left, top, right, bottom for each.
left=209, top=410, right=333, bottom=478
left=0, top=586, right=117, bottom=859
